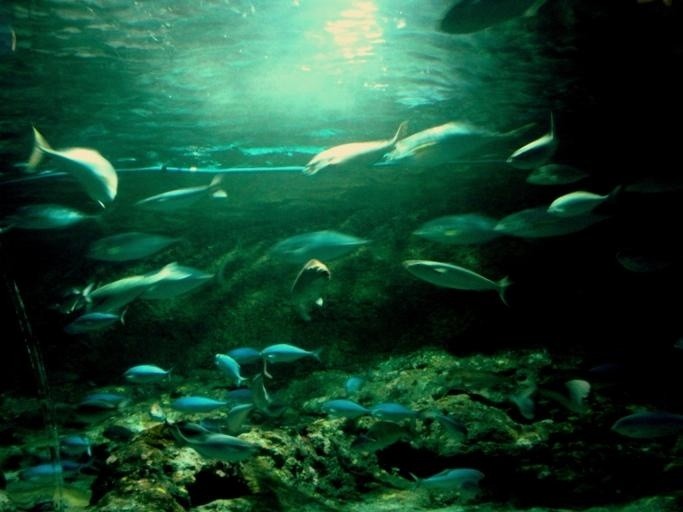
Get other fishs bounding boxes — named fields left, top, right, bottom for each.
left=0, top=109, right=620, bottom=501
